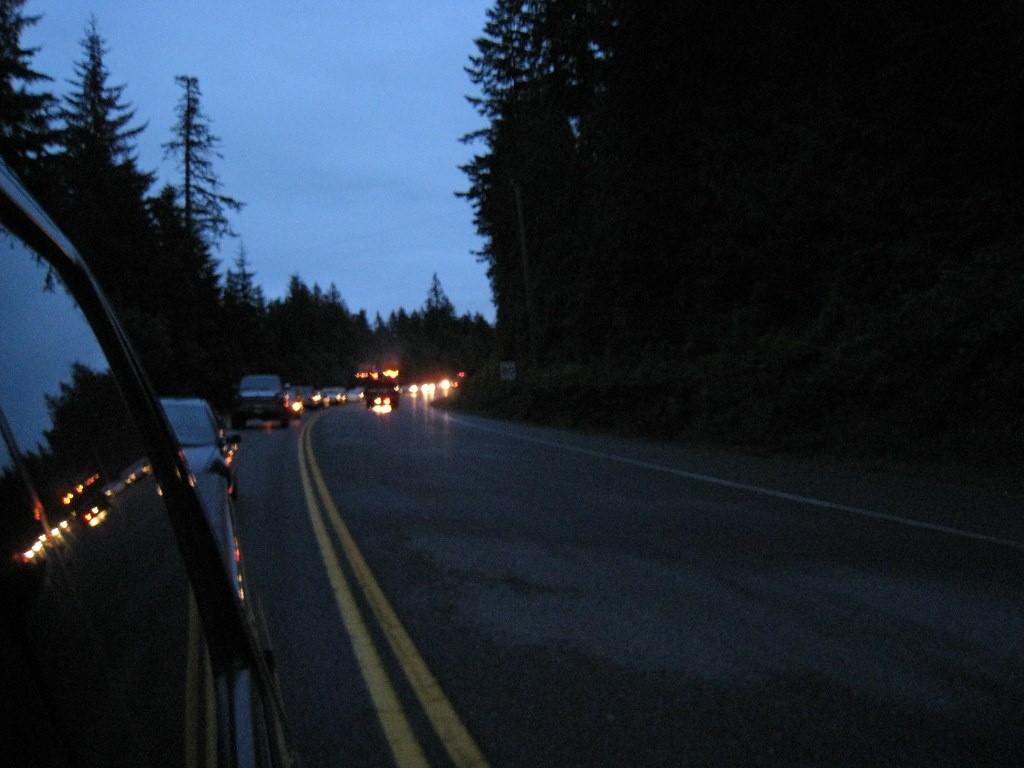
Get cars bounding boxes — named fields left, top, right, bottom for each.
left=0, top=147, right=457, bottom=768
